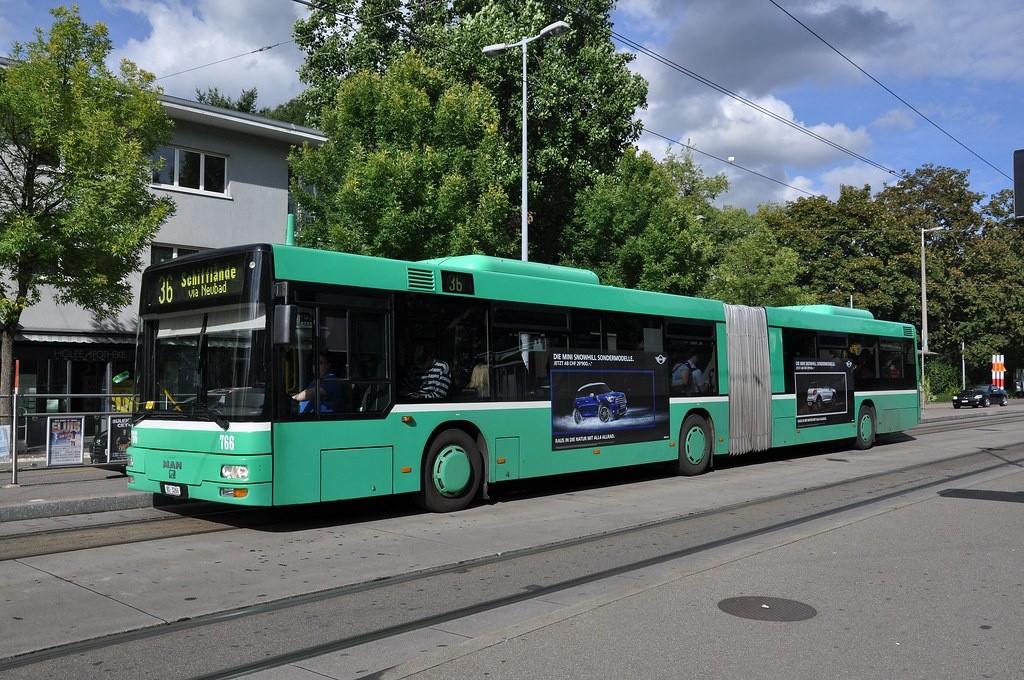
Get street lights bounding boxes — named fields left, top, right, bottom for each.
left=922, top=226, right=945, bottom=413
left=480, top=20, right=572, bottom=373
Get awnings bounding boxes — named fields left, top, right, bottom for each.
left=0, top=264, right=142, bottom=343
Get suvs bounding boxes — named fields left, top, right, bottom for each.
left=806, top=379, right=839, bottom=410
left=572, top=382, right=629, bottom=426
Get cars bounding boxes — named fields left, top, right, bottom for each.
left=88, top=387, right=265, bottom=478
left=952, top=383, right=1009, bottom=409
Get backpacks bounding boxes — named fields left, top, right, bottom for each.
left=669, top=361, right=699, bottom=394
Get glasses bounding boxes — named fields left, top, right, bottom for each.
left=312, top=361, right=323, bottom=365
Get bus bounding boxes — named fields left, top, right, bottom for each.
left=124, top=214, right=924, bottom=514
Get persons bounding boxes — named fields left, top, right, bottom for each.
left=402, top=345, right=451, bottom=399
left=672, top=351, right=710, bottom=394
left=464, top=364, right=488, bottom=397
left=292, top=351, right=335, bottom=411
left=887, top=366, right=900, bottom=378
left=855, top=357, right=872, bottom=379
